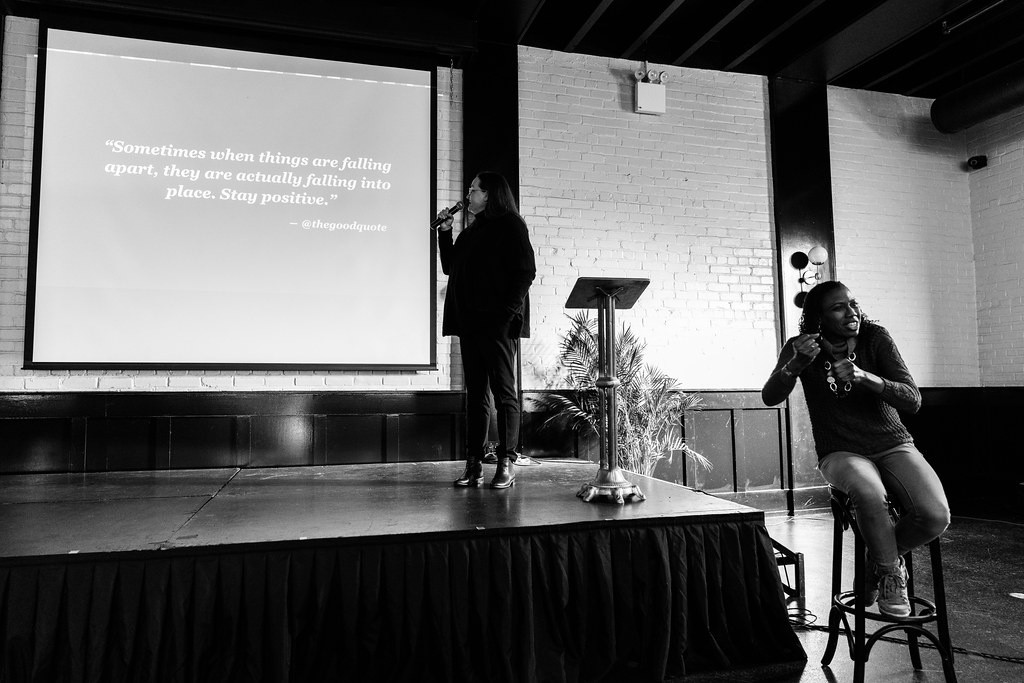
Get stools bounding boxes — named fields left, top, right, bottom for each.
left=821, top=485, right=956, bottom=683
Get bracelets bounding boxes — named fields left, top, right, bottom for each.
left=781, top=363, right=795, bottom=378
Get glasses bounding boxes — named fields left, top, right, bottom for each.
left=468, top=187, right=486, bottom=195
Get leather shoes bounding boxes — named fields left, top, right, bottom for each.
left=488, top=456, right=516, bottom=489
left=452, top=457, right=484, bottom=488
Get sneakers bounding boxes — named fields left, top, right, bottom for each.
left=872, top=554, right=912, bottom=618
left=851, top=557, right=879, bottom=607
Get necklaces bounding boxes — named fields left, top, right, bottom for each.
left=824, top=352, right=856, bottom=399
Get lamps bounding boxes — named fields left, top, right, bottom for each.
left=801, top=245, right=827, bottom=285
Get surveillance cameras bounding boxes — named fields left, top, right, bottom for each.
left=968, top=156, right=987, bottom=169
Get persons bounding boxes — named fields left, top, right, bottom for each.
left=763, top=281, right=950, bottom=620
left=437, top=173, right=536, bottom=489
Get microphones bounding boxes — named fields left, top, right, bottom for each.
left=430, top=201, right=464, bottom=231
